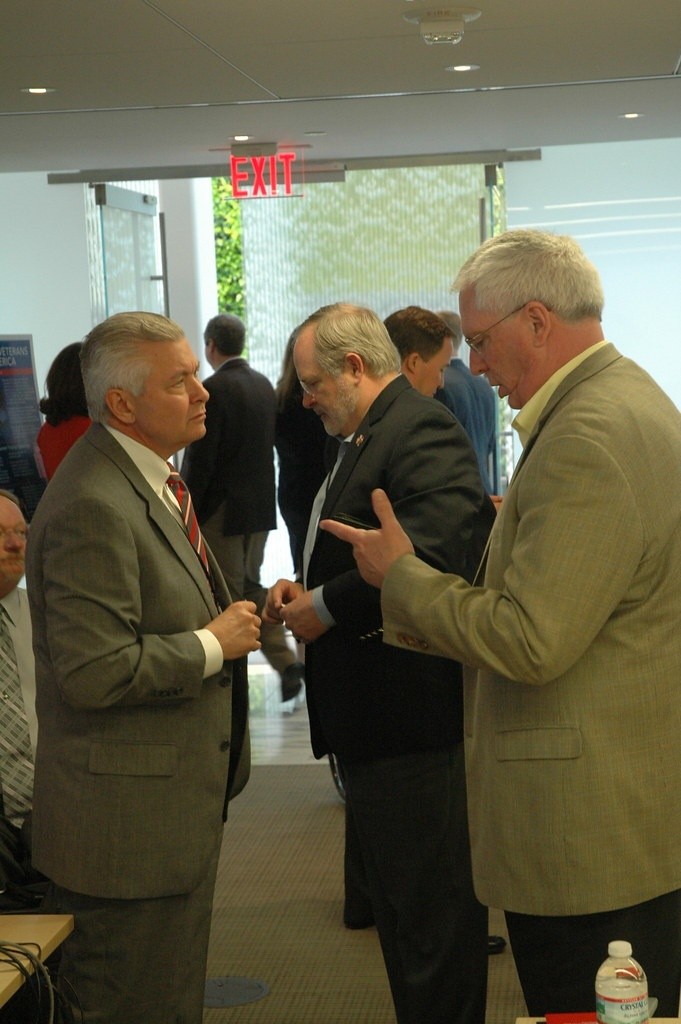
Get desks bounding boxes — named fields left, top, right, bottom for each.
left=0, top=915, right=76, bottom=1013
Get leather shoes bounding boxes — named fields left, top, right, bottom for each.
left=487, top=936, right=506, bottom=954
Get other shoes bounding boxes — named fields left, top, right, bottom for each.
left=280, top=659, right=304, bottom=701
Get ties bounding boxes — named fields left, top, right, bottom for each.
left=0, top=604, right=35, bottom=829
left=166, top=461, right=209, bottom=574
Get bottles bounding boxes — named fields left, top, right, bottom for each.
left=596, top=940, right=649, bottom=1024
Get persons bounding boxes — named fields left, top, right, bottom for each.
left=321, top=228, right=681, bottom=1018
left=262, top=302, right=498, bottom=1024
left=323, top=306, right=458, bottom=471
left=435, top=312, right=502, bottom=495
left=0, top=488, right=53, bottom=910
left=27, top=311, right=262, bottom=1024
left=181, top=314, right=306, bottom=703
left=36, top=344, right=93, bottom=481
left=274, top=325, right=326, bottom=665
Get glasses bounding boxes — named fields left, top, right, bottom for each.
left=299, top=359, right=342, bottom=397
left=465, top=302, right=552, bottom=353
